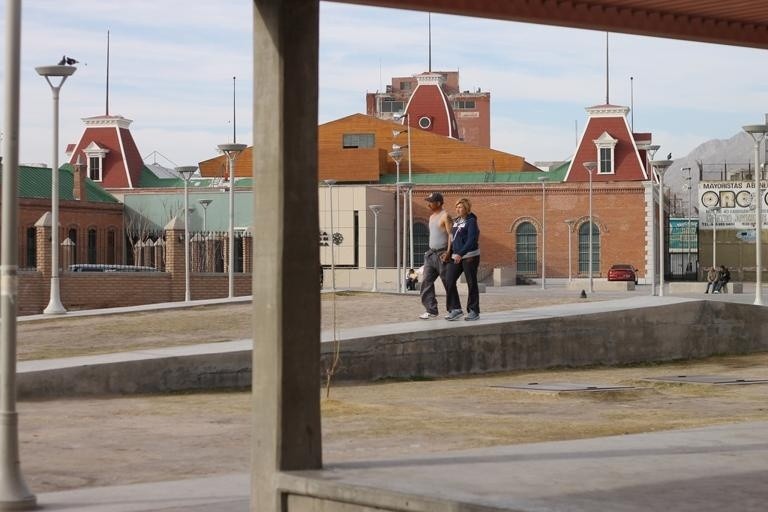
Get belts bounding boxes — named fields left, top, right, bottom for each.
left=429, top=246, right=448, bottom=253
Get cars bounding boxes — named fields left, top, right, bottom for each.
left=608, top=264, right=638, bottom=285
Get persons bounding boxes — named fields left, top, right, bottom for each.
left=705, top=267, right=719, bottom=293
left=711, top=264, right=731, bottom=294
left=417, top=192, right=456, bottom=320
left=439, top=197, right=481, bottom=322
left=406, top=269, right=419, bottom=290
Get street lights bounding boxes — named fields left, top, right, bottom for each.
left=538, top=176, right=548, bottom=288
left=583, top=162, right=597, bottom=292
left=370, top=204, right=384, bottom=293
left=176, top=166, right=198, bottom=301
left=564, top=219, right=575, bottom=284
left=710, top=207, right=721, bottom=266
left=645, top=145, right=673, bottom=297
left=218, top=144, right=247, bottom=298
left=35, top=67, right=76, bottom=314
left=198, top=200, right=212, bottom=231
left=742, top=125, right=768, bottom=305
left=389, top=150, right=414, bottom=294
left=324, top=179, right=337, bottom=288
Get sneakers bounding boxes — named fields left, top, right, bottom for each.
left=465, top=308, right=480, bottom=321
left=445, top=308, right=464, bottom=321
left=418, top=311, right=439, bottom=319
left=704, top=290, right=719, bottom=294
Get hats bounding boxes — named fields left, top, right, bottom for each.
left=424, top=192, right=443, bottom=202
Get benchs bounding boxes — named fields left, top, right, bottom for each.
left=570, top=280, right=635, bottom=291
left=402, top=280, right=486, bottom=294
left=669, top=282, right=744, bottom=295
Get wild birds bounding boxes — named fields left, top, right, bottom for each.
left=66, top=57, right=79, bottom=66
left=57, top=56, right=66, bottom=65
